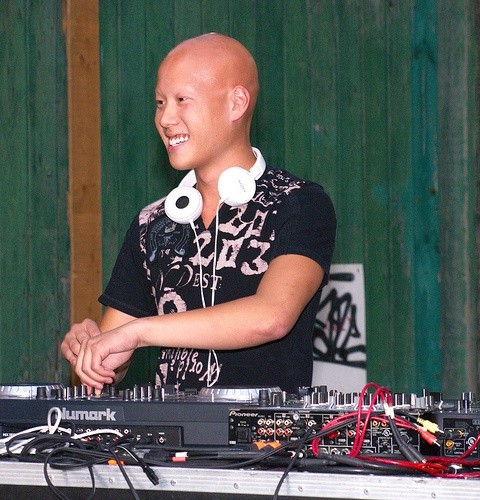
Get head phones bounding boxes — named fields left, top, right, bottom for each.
left=164, top=144, right=266, bottom=225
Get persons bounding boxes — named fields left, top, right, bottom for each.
left=64, top=31, right=340, bottom=396
